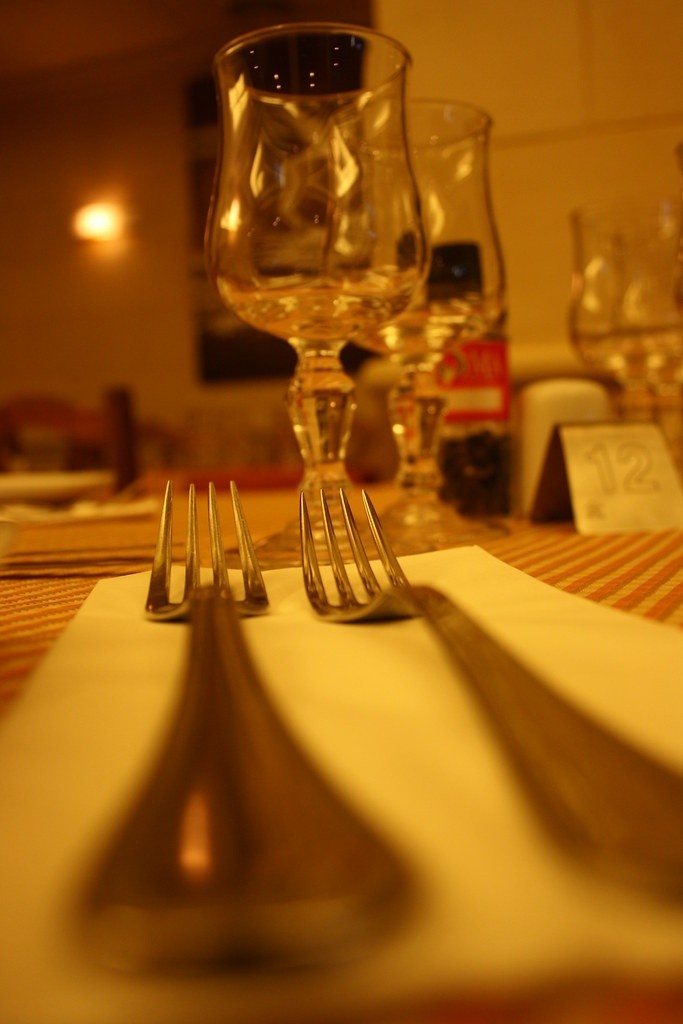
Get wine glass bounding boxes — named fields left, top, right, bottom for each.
left=206, top=22, right=432, bottom=564
left=328, top=99, right=507, bottom=555
left=569, top=198, right=683, bottom=465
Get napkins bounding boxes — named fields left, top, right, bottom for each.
left=0, top=545, right=683, bottom=1024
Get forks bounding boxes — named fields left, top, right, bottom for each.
left=297, top=491, right=683, bottom=903
left=75, top=480, right=416, bottom=982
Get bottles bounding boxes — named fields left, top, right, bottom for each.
left=431, top=240, right=508, bottom=515
